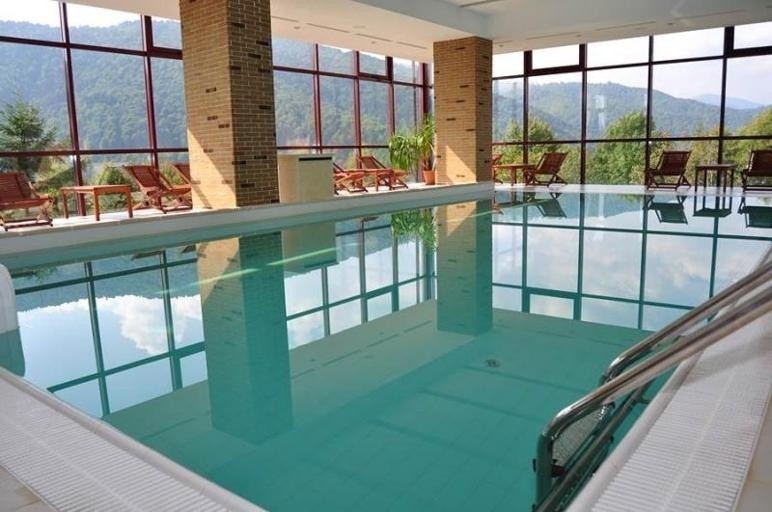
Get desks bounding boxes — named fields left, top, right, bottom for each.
left=696, top=164, right=738, bottom=191
left=494, top=163, right=535, bottom=185
left=61, top=184, right=133, bottom=221
left=692, top=196, right=733, bottom=218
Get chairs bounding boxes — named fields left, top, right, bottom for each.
left=0, top=171, right=54, bottom=233
left=517, top=192, right=567, bottom=219
left=740, top=149, right=772, bottom=192
left=120, top=161, right=191, bottom=214
left=525, top=151, right=570, bottom=187
left=332, top=156, right=408, bottom=195
left=645, top=150, right=693, bottom=192
left=737, top=197, right=772, bottom=229
left=643, top=195, right=688, bottom=224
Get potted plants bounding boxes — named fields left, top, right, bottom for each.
left=388, top=114, right=436, bottom=185
